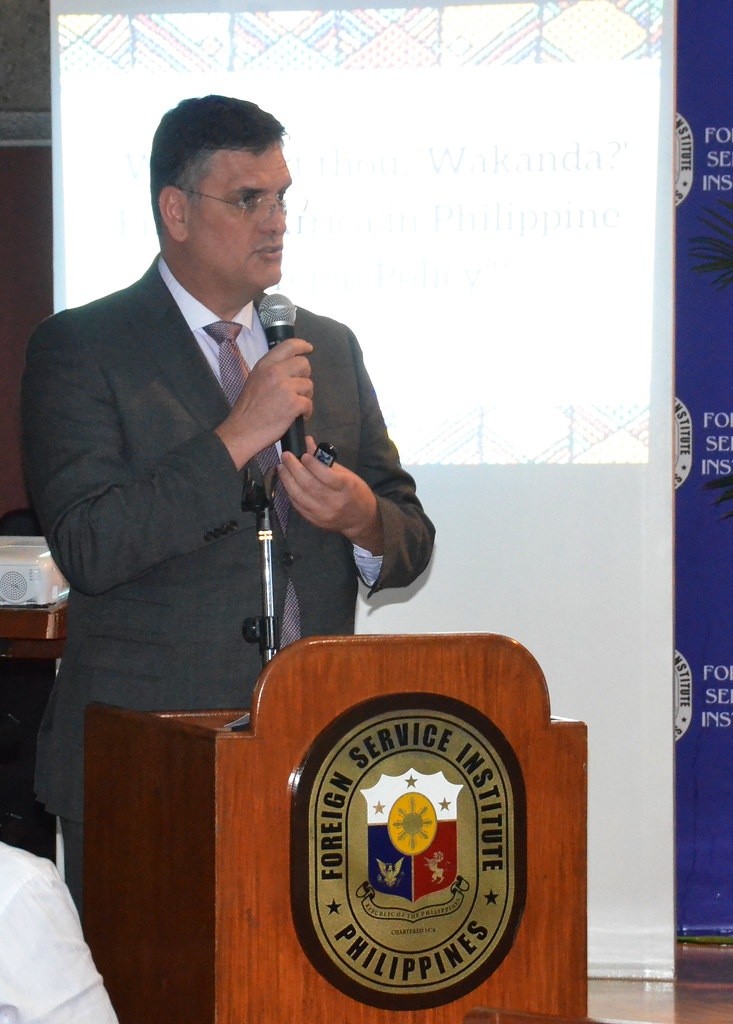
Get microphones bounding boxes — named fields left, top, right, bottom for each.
left=258, top=294, right=307, bottom=460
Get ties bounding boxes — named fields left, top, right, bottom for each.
left=202, top=321, right=301, bottom=650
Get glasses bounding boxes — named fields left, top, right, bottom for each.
left=178, top=186, right=308, bottom=223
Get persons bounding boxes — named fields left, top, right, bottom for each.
left=13, top=93, right=440, bottom=927
left=0, top=840, right=118, bottom=1024
